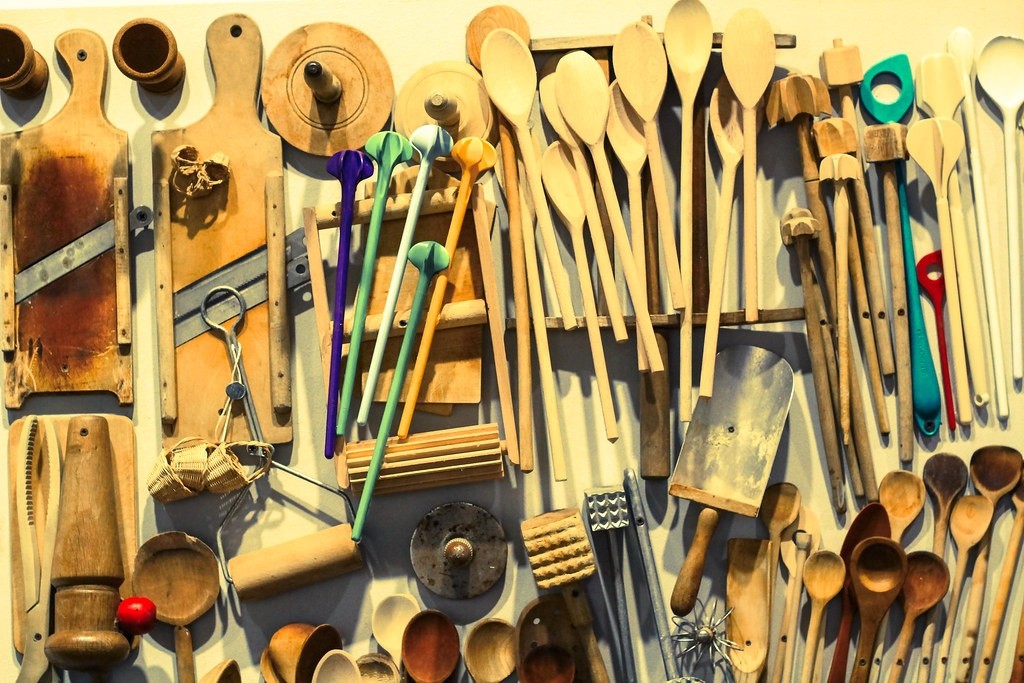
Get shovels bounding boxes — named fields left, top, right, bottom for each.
left=666, top=344, right=794, bottom=613
left=727, top=537, right=773, bottom=683
